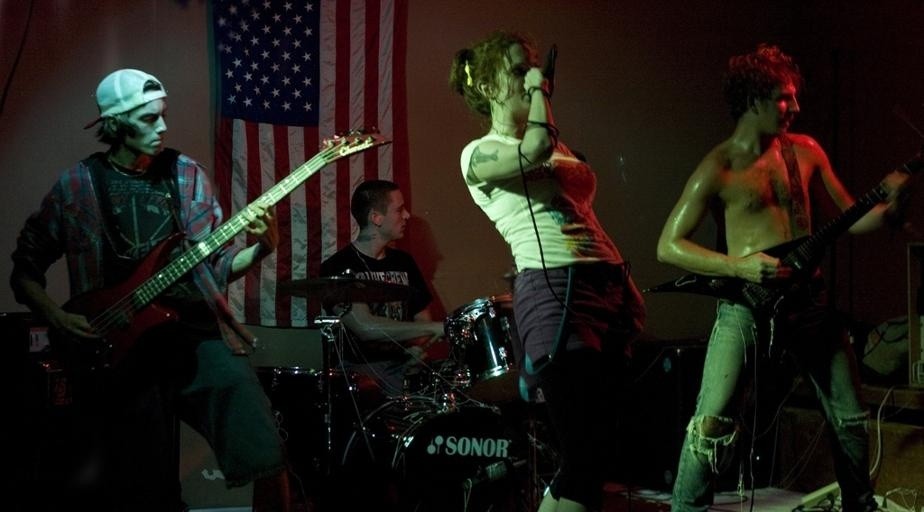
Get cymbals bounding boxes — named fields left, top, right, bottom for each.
left=278, top=275, right=422, bottom=303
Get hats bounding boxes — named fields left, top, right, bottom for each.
left=83, top=69, right=167, bottom=130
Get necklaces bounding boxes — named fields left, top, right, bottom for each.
left=353, top=247, right=383, bottom=285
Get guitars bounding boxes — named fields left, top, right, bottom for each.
left=640, top=151, right=923, bottom=365
left=46, top=126, right=394, bottom=376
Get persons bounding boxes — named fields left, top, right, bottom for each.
left=655, top=42, right=911, bottom=512
left=9, top=69, right=295, bottom=512
left=316, top=178, right=446, bottom=512
left=448, top=27, right=648, bottom=512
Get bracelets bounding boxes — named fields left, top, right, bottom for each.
left=527, top=85, right=549, bottom=103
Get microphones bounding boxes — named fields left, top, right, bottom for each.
left=542, top=43, right=558, bottom=99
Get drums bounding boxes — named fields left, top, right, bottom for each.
left=443, top=293, right=529, bottom=401
left=340, top=391, right=536, bottom=511
left=256, top=365, right=360, bottom=456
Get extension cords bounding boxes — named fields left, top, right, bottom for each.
left=801, top=479, right=842, bottom=507
